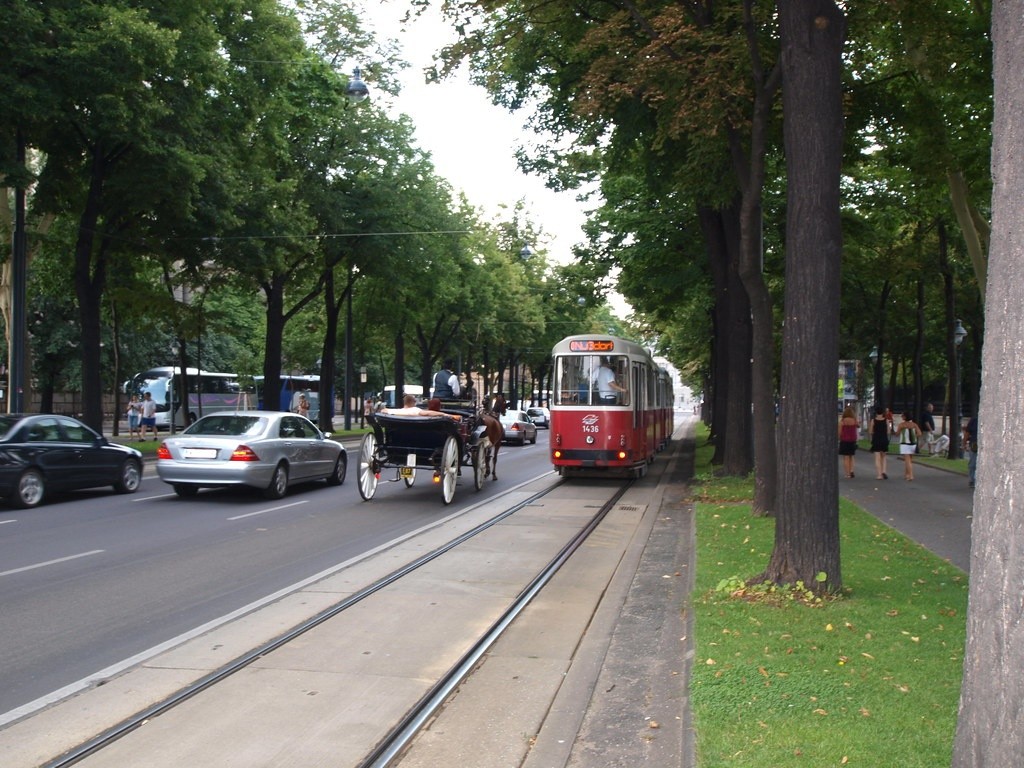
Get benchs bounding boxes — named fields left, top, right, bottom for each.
left=377, top=410, right=464, bottom=446
left=416, top=396, right=484, bottom=420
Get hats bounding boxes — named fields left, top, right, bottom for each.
left=442, top=357, right=454, bottom=363
left=299, top=393, right=305, bottom=396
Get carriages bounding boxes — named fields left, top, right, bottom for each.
left=356, top=394, right=509, bottom=506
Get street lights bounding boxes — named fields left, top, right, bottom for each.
left=0, top=124, right=49, bottom=414
left=319, top=66, right=370, bottom=430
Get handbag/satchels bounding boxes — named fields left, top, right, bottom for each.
left=971, top=443, right=977, bottom=452
left=364, top=408, right=370, bottom=416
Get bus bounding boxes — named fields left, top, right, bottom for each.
left=546, top=334, right=674, bottom=480
left=485, top=390, right=554, bottom=411
left=376, top=385, right=435, bottom=409
left=122, top=366, right=335, bottom=432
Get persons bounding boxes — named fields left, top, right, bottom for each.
left=869, top=407, right=895, bottom=479
left=896, top=409, right=921, bottom=481
left=296, top=393, right=310, bottom=421
left=432, top=359, right=461, bottom=401
left=917, top=403, right=935, bottom=454
left=576, top=356, right=628, bottom=405
left=838, top=406, right=861, bottom=479
left=363, top=398, right=387, bottom=428
left=962, top=411, right=978, bottom=487
left=381, top=396, right=461, bottom=422
left=127, top=392, right=158, bottom=442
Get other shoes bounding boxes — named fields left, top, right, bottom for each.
left=850, top=471, right=855, bottom=478
left=882, top=473, right=888, bottom=479
left=844, top=475, right=851, bottom=478
left=139, top=439, right=146, bottom=442
left=874, top=477, right=882, bottom=480
left=969, top=481, right=975, bottom=488
left=154, top=437, right=158, bottom=441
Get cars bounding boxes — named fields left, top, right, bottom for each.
left=156, top=411, right=348, bottom=501
left=0, top=414, right=145, bottom=510
left=499, top=409, right=538, bottom=447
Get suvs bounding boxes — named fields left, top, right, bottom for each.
left=526, top=407, right=551, bottom=429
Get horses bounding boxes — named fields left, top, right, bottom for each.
left=471, top=393, right=506, bottom=482
